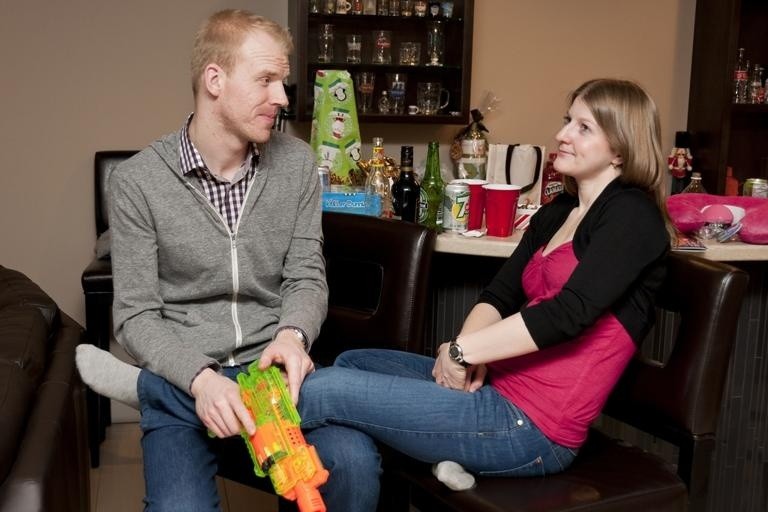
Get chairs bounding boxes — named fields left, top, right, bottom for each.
left=394, top=250, right=743, bottom=512
left=216, top=214, right=436, bottom=512
left=81, top=150, right=140, bottom=467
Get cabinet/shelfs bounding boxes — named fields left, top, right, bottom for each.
left=287, top=1, right=475, bottom=124
left=687, top=1, right=768, bottom=195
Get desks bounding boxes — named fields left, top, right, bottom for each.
left=410, top=228, right=767, bottom=510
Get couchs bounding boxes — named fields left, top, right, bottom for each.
left=0, top=265, right=89, bottom=510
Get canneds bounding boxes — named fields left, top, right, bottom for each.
left=317, top=165, right=332, bottom=193
left=442, top=182, right=470, bottom=235
left=742, top=178, right=768, bottom=200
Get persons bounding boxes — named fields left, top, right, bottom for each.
left=106, top=9, right=383, bottom=511
left=73, top=76, right=674, bottom=480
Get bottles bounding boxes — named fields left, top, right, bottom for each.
left=538, top=152, right=565, bottom=207
left=418, top=140, right=445, bottom=234
left=667, top=130, right=697, bottom=195
left=456, top=129, right=489, bottom=182
left=724, top=167, right=738, bottom=195
left=390, top=144, right=421, bottom=223
left=679, top=171, right=707, bottom=196
left=425, top=22, right=446, bottom=65
left=377, top=91, right=389, bottom=112
left=733, top=47, right=768, bottom=103
left=365, top=135, right=391, bottom=221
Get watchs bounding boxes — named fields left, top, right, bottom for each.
left=447, top=334, right=473, bottom=370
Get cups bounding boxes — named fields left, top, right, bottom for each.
left=371, top=29, right=392, bottom=63
left=481, top=185, right=521, bottom=237
left=417, top=82, right=450, bottom=114
left=454, top=179, right=489, bottom=230
left=352, top=71, right=376, bottom=112
left=407, top=105, right=418, bottom=115
left=309, top=1, right=456, bottom=21
left=318, top=24, right=336, bottom=63
left=395, top=42, right=422, bottom=65
left=385, top=71, right=406, bottom=114
left=346, top=34, right=362, bottom=63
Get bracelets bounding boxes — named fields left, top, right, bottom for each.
left=276, top=325, right=311, bottom=353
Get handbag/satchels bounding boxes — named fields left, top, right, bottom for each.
left=487, top=143, right=545, bottom=209
